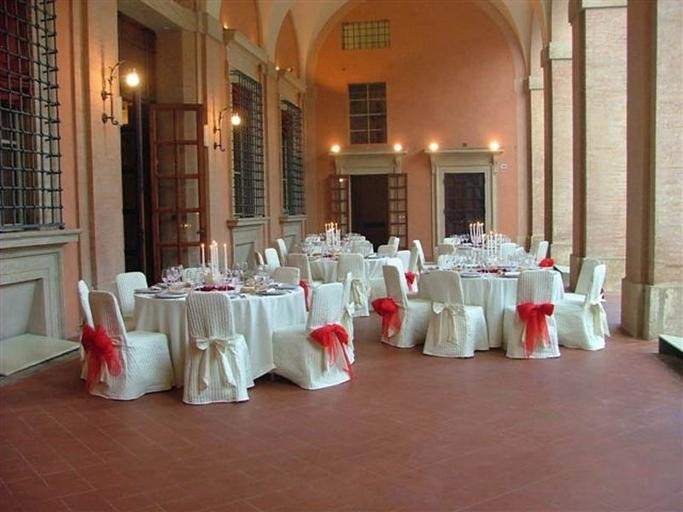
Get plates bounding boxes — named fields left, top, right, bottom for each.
left=155, top=293, right=188, bottom=299
left=134, top=288, right=161, bottom=293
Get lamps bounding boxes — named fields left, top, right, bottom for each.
left=101, top=58, right=139, bottom=125
left=214, top=107, right=241, bottom=151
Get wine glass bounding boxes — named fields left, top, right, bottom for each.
left=161, top=261, right=271, bottom=291
left=443, top=254, right=520, bottom=274
left=449, top=233, right=470, bottom=244
